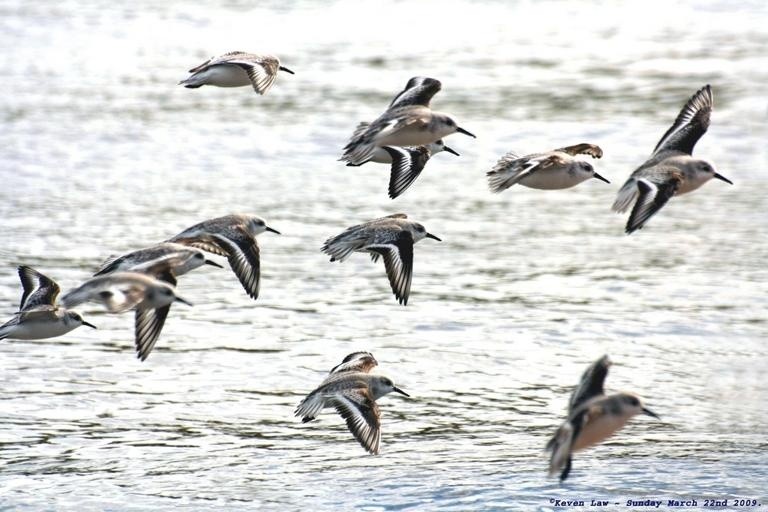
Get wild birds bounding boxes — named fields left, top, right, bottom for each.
left=609, top=81, right=734, bottom=234
left=294, top=350, right=410, bottom=456
left=1, top=265, right=95, bottom=341
left=320, top=213, right=442, bottom=308
left=56, top=212, right=281, bottom=364
left=486, top=143, right=610, bottom=193
left=178, top=50, right=297, bottom=97
left=337, top=76, right=478, bottom=200
left=542, top=352, right=662, bottom=486
